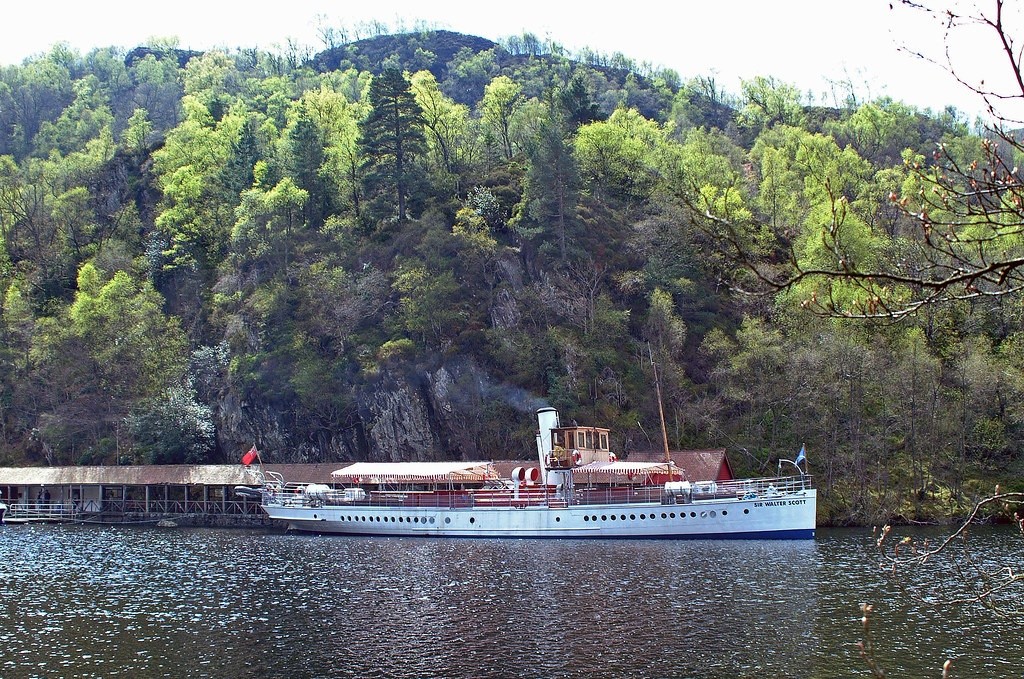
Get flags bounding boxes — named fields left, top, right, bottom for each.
left=794, top=446, right=805, bottom=467
left=243, top=446, right=257, bottom=466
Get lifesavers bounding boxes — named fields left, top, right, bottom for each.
left=294, top=485, right=307, bottom=494
left=609, top=451, right=617, bottom=463
left=572, top=449, right=581, bottom=465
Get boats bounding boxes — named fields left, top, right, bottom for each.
left=233, top=484, right=263, bottom=500
left=254, top=363, right=819, bottom=543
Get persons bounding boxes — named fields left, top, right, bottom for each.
left=36, top=490, right=51, bottom=509
